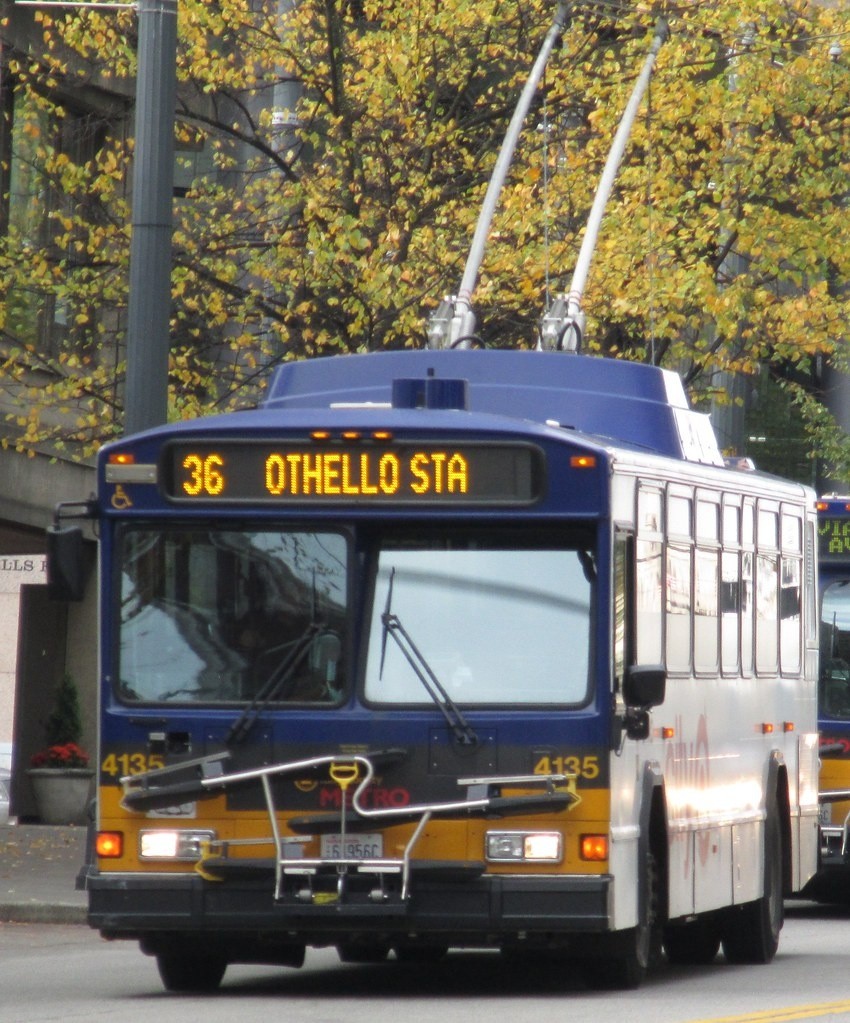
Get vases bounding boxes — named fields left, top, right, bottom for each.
left=25, top=768, right=96, bottom=825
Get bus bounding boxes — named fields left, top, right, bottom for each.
left=44, top=23, right=827, bottom=997
left=810, top=491, right=850, bottom=911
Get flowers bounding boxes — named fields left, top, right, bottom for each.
left=31, top=744, right=90, bottom=768
